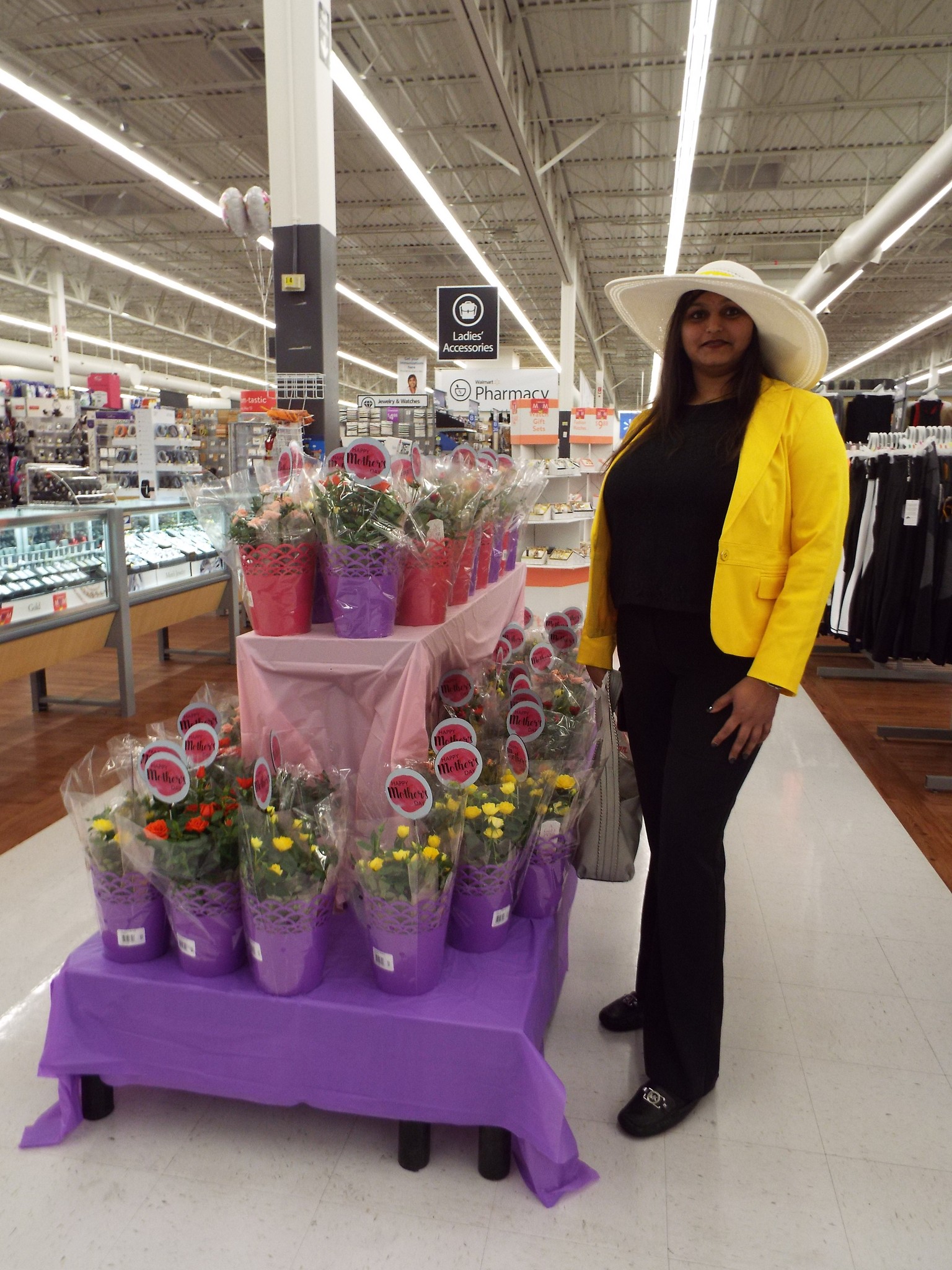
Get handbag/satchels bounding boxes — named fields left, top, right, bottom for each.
left=569, top=670, right=643, bottom=882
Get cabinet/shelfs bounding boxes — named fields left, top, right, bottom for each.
left=0, top=495, right=238, bottom=718
left=507, top=399, right=613, bottom=587
left=5, top=407, right=306, bottom=495
left=25, top=462, right=121, bottom=509
left=347, top=405, right=512, bottom=458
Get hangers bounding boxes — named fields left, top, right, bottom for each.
left=846, top=422, right=952, bottom=460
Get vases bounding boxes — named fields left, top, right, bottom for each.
left=468, top=525, right=482, bottom=596
left=241, top=875, right=333, bottom=997
left=488, top=520, right=504, bottom=583
left=152, top=859, right=250, bottom=975
left=499, top=523, right=510, bottom=577
left=446, top=850, right=521, bottom=953
left=395, top=537, right=449, bottom=624
left=89, top=858, right=165, bottom=960
left=435, top=530, right=475, bottom=607
left=475, top=520, right=494, bottom=590
left=235, top=542, right=313, bottom=636
left=357, top=873, right=445, bottom=994
left=510, top=826, right=576, bottom=919
left=319, top=536, right=400, bottom=640
left=505, top=528, right=518, bottom=572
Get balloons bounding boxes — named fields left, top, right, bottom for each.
left=243, top=185, right=271, bottom=231
left=220, top=187, right=249, bottom=238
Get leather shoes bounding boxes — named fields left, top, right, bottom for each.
left=599, top=989, right=718, bottom=1137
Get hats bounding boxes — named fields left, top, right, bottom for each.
left=602, top=260, right=829, bottom=391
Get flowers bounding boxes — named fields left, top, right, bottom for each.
left=352, top=819, right=451, bottom=902
left=135, top=765, right=260, bottom=881
left=84, top=785, right=153, bottom=874
left=229, top=458, right=526, bottom=536
left=177, top=697, right=330, bottom=803
left=245, top=805, right=335, bottom=896
left=422, top=615, right=604, bottom=854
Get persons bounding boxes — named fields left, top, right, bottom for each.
left=575, top=260, right=854, bottom=1139
left=403, top=374, right=423, bottom=393
